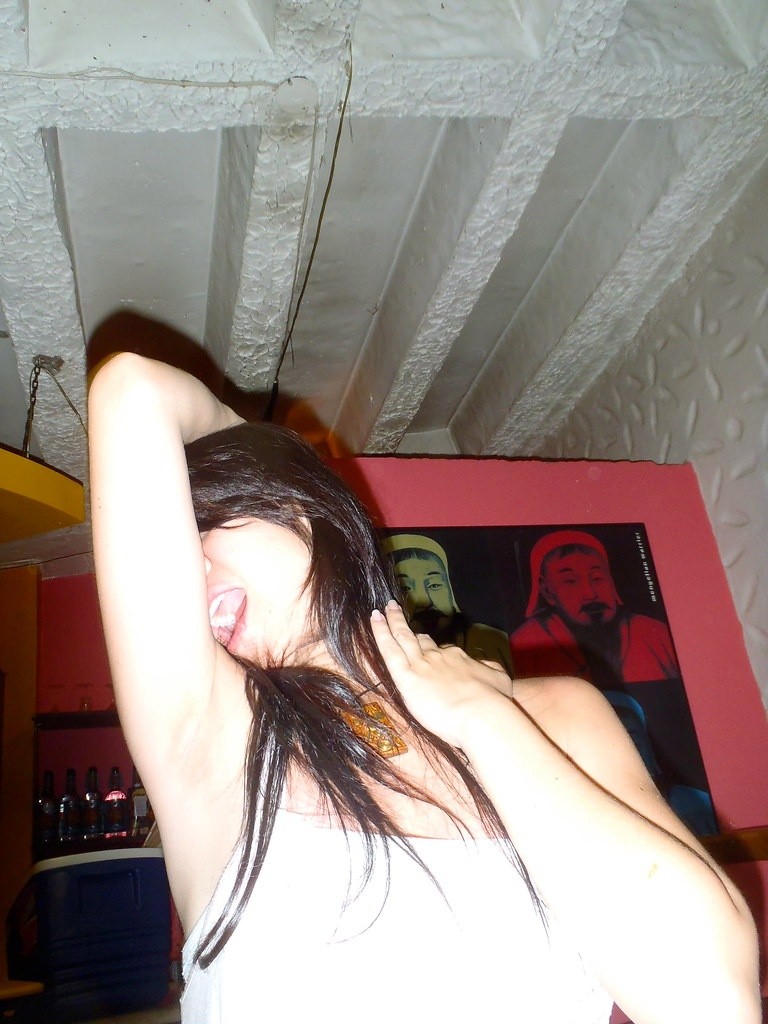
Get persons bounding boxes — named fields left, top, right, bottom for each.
left=86, top=349, right=763, bottom=1024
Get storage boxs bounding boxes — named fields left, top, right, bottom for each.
left=5, top=848, right=172, bottom=1024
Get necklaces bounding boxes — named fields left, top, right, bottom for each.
left=334, top=703, right=407, bottom=763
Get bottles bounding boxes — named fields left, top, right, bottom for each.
left=35, top=770, right=58, bottom=861
left=58, top=769, right=82, bottom=855
left=102, top=767, right=129, bottom=849
left=128, top=766, right=155, bottom=848
left=81, top=767, right=103, bottom=852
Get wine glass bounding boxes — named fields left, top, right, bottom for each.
left=46, top=683, right=117, bottom=711
